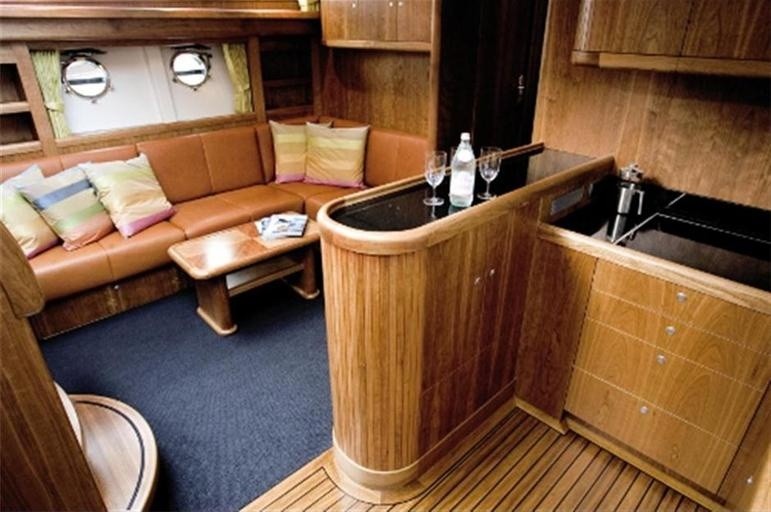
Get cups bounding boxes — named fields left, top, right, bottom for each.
left=422, top=151, right=447, bottom=206
left=476, top=147, right=503, bottom=200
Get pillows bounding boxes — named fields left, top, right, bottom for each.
left=78, top=154, right=176, bottom=240
left=14, top=160, right=115, bottom=253
left=0, top=163, right=60, bottom=260
left=303, top=122, right=370, bottom=189
left=269, top=120, right=333, bottom=185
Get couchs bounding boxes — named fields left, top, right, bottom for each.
left=0, top=114, right=426, bottom=343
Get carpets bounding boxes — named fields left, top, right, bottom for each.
left=38, top=286, right=333, bottom=512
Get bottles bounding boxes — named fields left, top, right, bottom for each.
left=448, top=132, right=476, bottom=208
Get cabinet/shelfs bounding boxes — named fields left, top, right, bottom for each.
left=319, top=1, right=440, bottom=52
left=569, top=0, right=771, bottom=79
left=516, top=140, right=771, bottom=512
left=320, top=192, right=536, bottom=488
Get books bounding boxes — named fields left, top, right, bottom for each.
left=253, top=214, right=310, bottom=237
